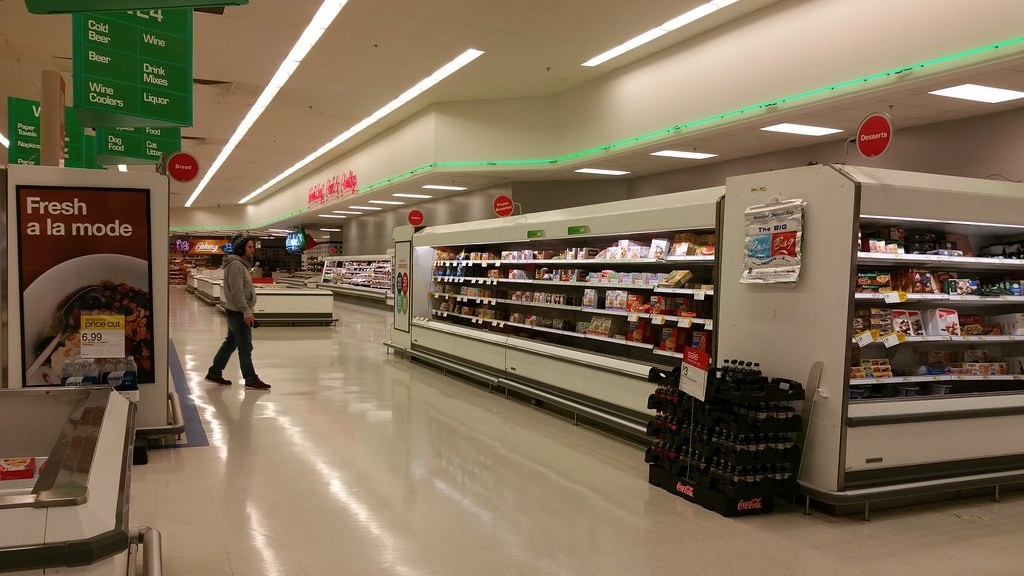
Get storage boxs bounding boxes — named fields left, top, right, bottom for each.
left=430, top=232, right=718, bottom=357
left=849, top=222, right=1023, bottom=398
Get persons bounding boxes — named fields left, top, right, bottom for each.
left=204, top=232, right=271, bottom=390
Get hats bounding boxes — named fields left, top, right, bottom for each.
left=230, top=234, right=250, bottom=252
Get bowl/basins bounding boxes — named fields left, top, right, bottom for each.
left=22, top=253, right=149, bottom=385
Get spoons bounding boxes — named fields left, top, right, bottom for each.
left=24, top=286, right=104, bottom=380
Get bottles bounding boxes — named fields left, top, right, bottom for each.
left=650, top=436, right=793, bottom=486
left=720, top=357, right=762, bottom=395
left=655, top=383, right=796, bottom=426
left=59, top=352, right=138, bottom=388
left=652, top=410, right=794, bottom=455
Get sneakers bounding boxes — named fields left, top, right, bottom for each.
left=204, top=373, right=232, bottom=386
left=244, top=379, right=271, bottom=390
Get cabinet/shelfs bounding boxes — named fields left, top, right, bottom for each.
left=185, top=270, right=223, bottom=293
left=316, top=255, right=395, bottom=306
left=849, top=250, right=1024, bottom=403
left=427, top=249, right=711, bottom=376
left=215, top=282, right=338, bottom=326
left=278, top=271, right=322, bottom=288
left=194, top=277, right=288, bottom=305
left=168, top=253, right=216, bottom=285
left=645, top=367, right=805, bottom=517
